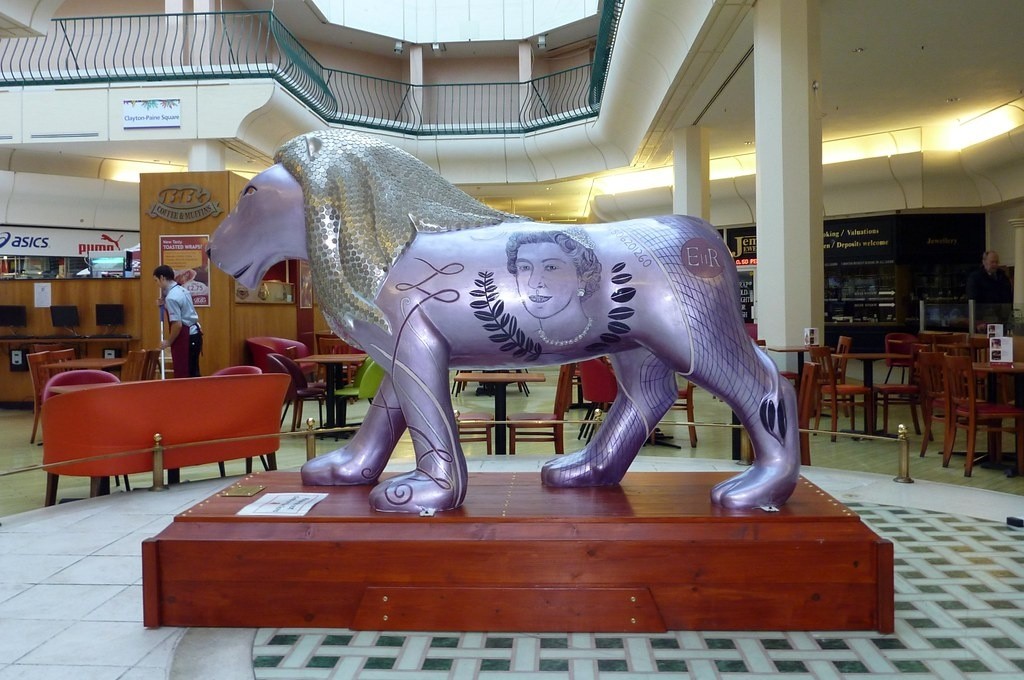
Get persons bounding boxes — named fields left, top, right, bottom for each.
left=965, top=250, right=1013, bottom=332
left=152, top=265, right=204, bottom=378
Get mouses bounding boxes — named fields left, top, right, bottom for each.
left=80, top=336, right=85, bottom=339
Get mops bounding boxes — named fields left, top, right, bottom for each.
left=158, top=287, right=167, bottom=379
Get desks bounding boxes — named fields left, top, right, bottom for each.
left=454, top=374, right=547, bottom=454
left=767, top=346, right=836, bottom=384
left=40, top=358, right=128, bottom=373
left=889, top=340, right=971, bottom=390
left=832, top=353, right=912, bottom=442
left=292, top=354, right=367, bottom=436
left=971, top=360, right=1024, bottom=460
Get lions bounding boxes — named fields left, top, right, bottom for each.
left=204, top=131, right=799, bottom=514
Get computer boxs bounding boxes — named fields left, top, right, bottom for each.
left=9, top=349, right=29, bottom=372
left=102, top=348, right=122, bottom=371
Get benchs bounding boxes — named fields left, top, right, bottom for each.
left=40, top=370, right=296, bottom=504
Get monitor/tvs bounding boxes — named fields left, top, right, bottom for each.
left=50, top=306, right=80, bottom=327
left=95, top=303, right=124, bottom=325
left=0, top=305, right=27, bottom=327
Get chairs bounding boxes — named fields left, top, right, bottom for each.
left=740, top=325, right=1024, bottom=476
left=27, top=334, right=697, bottom=507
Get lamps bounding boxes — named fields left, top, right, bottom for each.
left=395, top=41, right=403, bottom=55
left=434, top=43, right=442, bottom=56
left=538, top=35, right=547, bottom=48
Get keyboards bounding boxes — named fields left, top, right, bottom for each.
left=36, top=335, right=81, bottom=339
left=88, top=335, right=132, bottom=339
left=0, top=336, right=32, bottom=339
